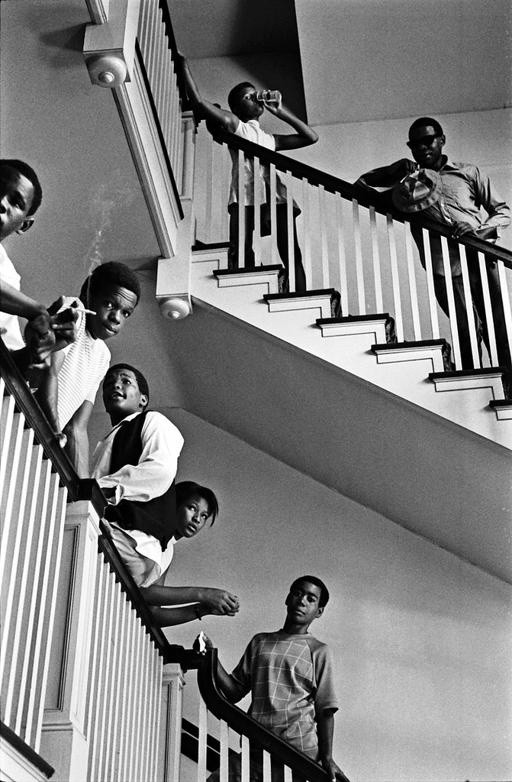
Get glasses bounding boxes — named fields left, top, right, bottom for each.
left=407, top=133, right=438, bottom=148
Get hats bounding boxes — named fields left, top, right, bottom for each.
left=393, top=168, right=442, bottom=214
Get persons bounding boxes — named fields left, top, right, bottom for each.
left=137, top=480, right=241, bottom=629
left=192, top=573, right=349, bottom=782
left=352, top=116, right=512, bottom=404
left=2, top=157, right=83, bottom=367
left=90, top=361, right=186, bottom=588
left=177, top=49, right=320, bottom=295
left=24, top=261, right=143, bottom=479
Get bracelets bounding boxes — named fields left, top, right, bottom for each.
left=194, top=601, right=203, bottom=621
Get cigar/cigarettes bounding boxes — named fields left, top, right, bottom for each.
left=61, top=304, right=97, bottom=316
